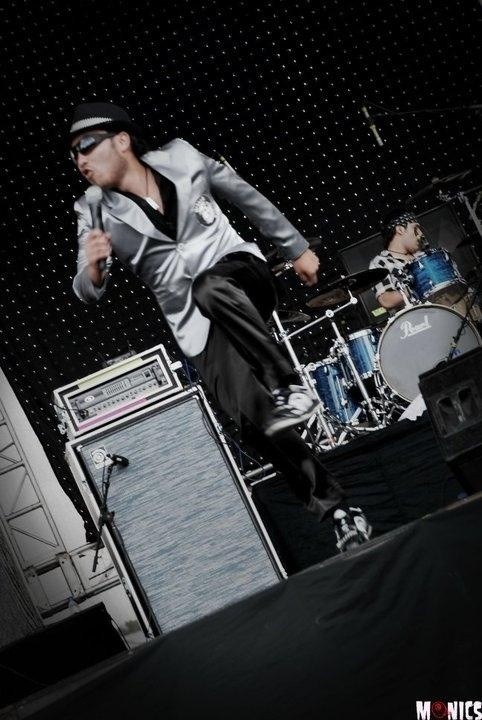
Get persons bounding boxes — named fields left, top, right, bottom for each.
left=64, top=97, right=373, bottom=560
left=364, top=204, right=482, bottom=334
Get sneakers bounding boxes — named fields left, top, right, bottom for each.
left=332, top=506, right=372, bottom=552
left=262, top=383, right=321, bottom=436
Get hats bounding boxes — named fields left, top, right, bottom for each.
left=67, top=102, right=130, bottom=147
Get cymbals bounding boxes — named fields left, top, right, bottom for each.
left=305, top=268, right=390, bottom=311
left=266, top=309, right=311, bottom=326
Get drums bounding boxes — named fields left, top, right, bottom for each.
left=375, top=300, right=482, bottom=403
left=403, top=246, right=469, bottom=307
left=329, top=329, right=380, bottom=390
left=302, top=356, right=366, bottom=436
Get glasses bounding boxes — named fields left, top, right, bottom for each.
left=68, top=133, right=117, bottom=164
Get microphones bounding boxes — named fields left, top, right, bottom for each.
left=413, top=229, right=432, bottom=257
left=86, top=185, right=107, bottom=271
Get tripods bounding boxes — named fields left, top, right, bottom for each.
left=268, top=299, right=409, bottom=454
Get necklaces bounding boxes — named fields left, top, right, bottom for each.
left=388, top=249, right=408, bottom=256
left=140, top=165, right=160, bottom=213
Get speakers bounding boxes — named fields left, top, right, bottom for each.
left=1, top=601, right=132, bottom=707
left=65, top=381, right=289, bottom=636
left=418, top=343, right=482, bottom=496
